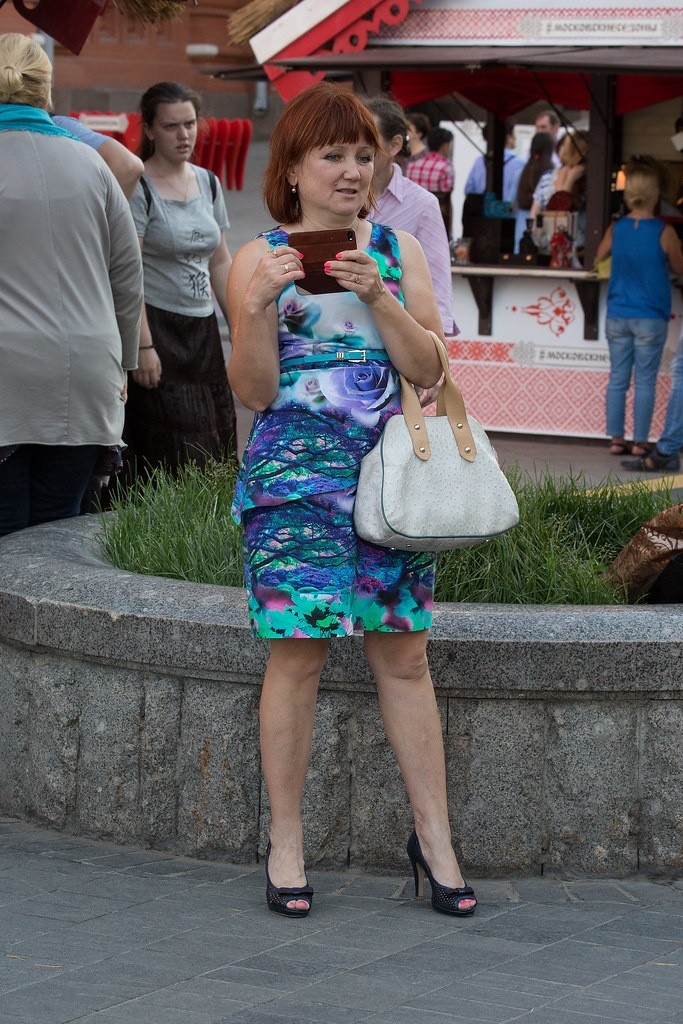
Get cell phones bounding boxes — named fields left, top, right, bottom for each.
left=288, top=229, right=357, bottom=272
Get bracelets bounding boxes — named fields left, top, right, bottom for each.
left=139, top=345, right=157, bottom=349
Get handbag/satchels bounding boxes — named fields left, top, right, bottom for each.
left=610, top=503, right=683, bottom=604
left=352, top=331, right=519, bottom=549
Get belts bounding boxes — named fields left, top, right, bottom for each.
left=281, top=349, right=390, bottom=366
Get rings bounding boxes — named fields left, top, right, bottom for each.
left=355, top=274, right=361, bottom=284
left=271, top=249, right=278, bottom=257
left=284, top=265, right=289, bottom=272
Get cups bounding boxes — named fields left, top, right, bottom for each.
left=447, top=238, right=470, bottom=265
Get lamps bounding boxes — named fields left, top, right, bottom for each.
left=185, top=43, right=221, bottom=80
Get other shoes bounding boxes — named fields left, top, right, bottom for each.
left=620, top=450, right=680, bottom=472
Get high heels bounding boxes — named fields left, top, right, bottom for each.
left=265, top=838, right=314, bottom=918
left=406, top=829, right=478, bottom=917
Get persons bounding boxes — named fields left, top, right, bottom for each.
left=357, top=91, right=453, bottom=335
left=120, top=81, right=232, bottom=505
left=0, top=33, right=143, bottom=540
left=596, top=167, right=683, bottom=458
left=46, top=111, right=145, bottom=199
left=619, top=330, right=683, bottom=473
left=528, top=130, right=585, bottom=249
left=427, top=126, right=454, bottom=158
left=402, top=114, right=454, bottom=241
left=513, top=132, right=554, bottom=252
left=462, top=117, right=529, bottom=254
left=536, top=110, right=562, bottom=171
left=608, top=155, right=664, bottom=217
left=225, top=84, right=478, bottom=918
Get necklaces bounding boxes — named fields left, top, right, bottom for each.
left=149, top=159, right=193, bottom=201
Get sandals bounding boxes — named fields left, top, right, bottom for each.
left=608, top=443, right=630, bottom=456
left=631, top=442, right=654, bottom=456
